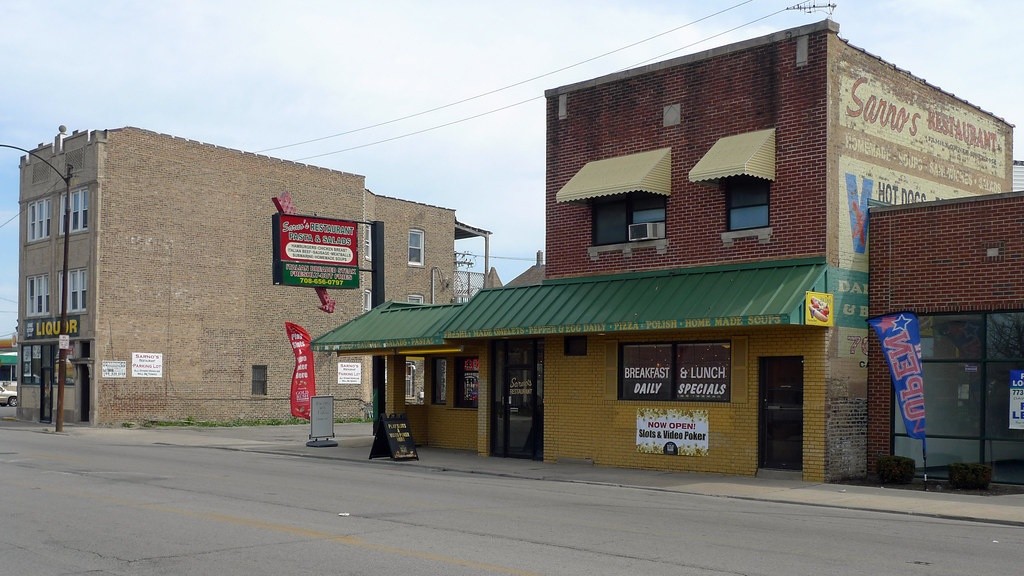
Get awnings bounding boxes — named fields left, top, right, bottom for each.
left=556, top=147, right=671, bottom=204
left=689, top=128, right=775, bottom=182
left=310, top=303, right=463, bottom=350
left=440, top=257, right=827, bottom=339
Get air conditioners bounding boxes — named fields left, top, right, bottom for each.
left=628, top=223, right=658, bottom=242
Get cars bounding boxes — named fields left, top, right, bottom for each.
left=0, top=386, right=17, bottom=406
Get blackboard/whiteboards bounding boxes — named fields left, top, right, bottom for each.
left=383, top=418, right=418, bottom=459
left=310, top=396, right=334, bottom=438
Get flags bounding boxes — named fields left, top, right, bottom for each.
left=870, top=312, right=925, bottom=440
left=284, top=322, right=316, bottom=419
